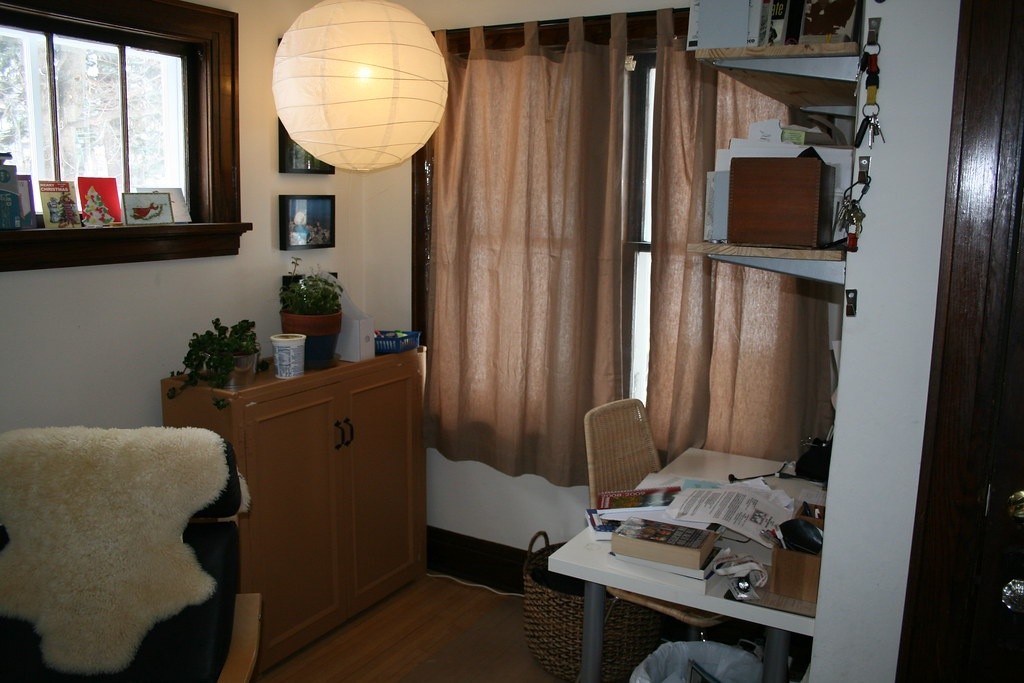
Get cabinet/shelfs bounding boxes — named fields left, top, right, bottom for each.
left=684, top=45, right=859, bottom=283
left=242, top=358, right=420, bottom=657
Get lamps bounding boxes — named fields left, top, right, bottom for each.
left=272, top=0, right=449, bottom=170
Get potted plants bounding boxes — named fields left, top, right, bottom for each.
left=167, top=317, right=269, bottom=409
left=278, top=255, right=344, bottom=368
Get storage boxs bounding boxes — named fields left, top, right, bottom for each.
left=769, top=502, right=825, bottom=620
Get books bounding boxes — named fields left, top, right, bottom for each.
left=585, top=478, right=731, bottom=595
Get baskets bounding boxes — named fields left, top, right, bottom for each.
left=523, top=530, right=668, bottom=683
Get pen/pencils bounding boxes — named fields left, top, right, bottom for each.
left=803, top=501, right=821, bottom=518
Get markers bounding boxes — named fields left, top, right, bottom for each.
left=374, top=328, right=385, bottom=337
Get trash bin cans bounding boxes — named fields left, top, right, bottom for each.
left=629, top=639, right=764, bottom=683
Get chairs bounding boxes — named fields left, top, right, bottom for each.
left=576, top=398, right=732, bottom=683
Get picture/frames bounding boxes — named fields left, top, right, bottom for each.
left=122, top=192, right=174, bottom=226
left=277, top=117, right=335, bottom=175
left=279, top=195, right=335, bottom=251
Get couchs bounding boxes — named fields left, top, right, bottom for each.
left=0, top=426, right=265, bottom=683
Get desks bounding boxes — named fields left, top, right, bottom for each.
left=549, top=447, right=829, bottom=683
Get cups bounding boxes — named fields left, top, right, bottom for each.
left=271, top=334, right=306, bottom=381
